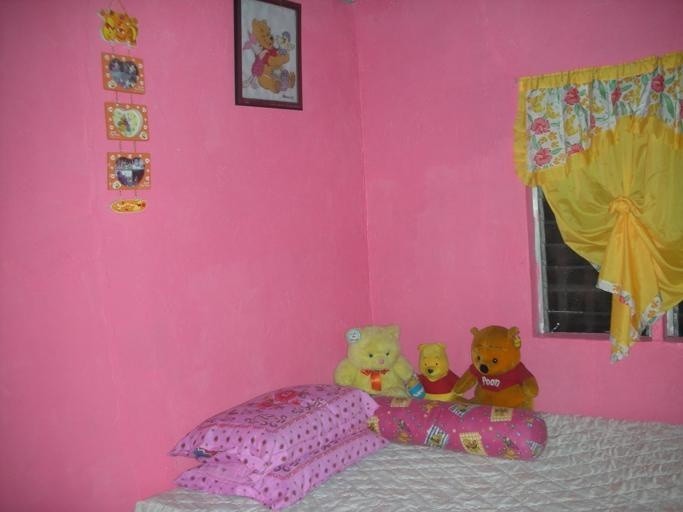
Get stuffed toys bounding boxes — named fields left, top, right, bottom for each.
left=334, top=325, right=425, bottom=400
left=413, top=341, right=465, bottom=401
left=446, top=325, right=538, bottom=411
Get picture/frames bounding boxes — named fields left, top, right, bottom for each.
left=234, top=1, right=302, bottom=110
left=102, top=54, right=151, bottom=190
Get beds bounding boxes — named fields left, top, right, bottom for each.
left=135, top=413, right=682, bottom=512
